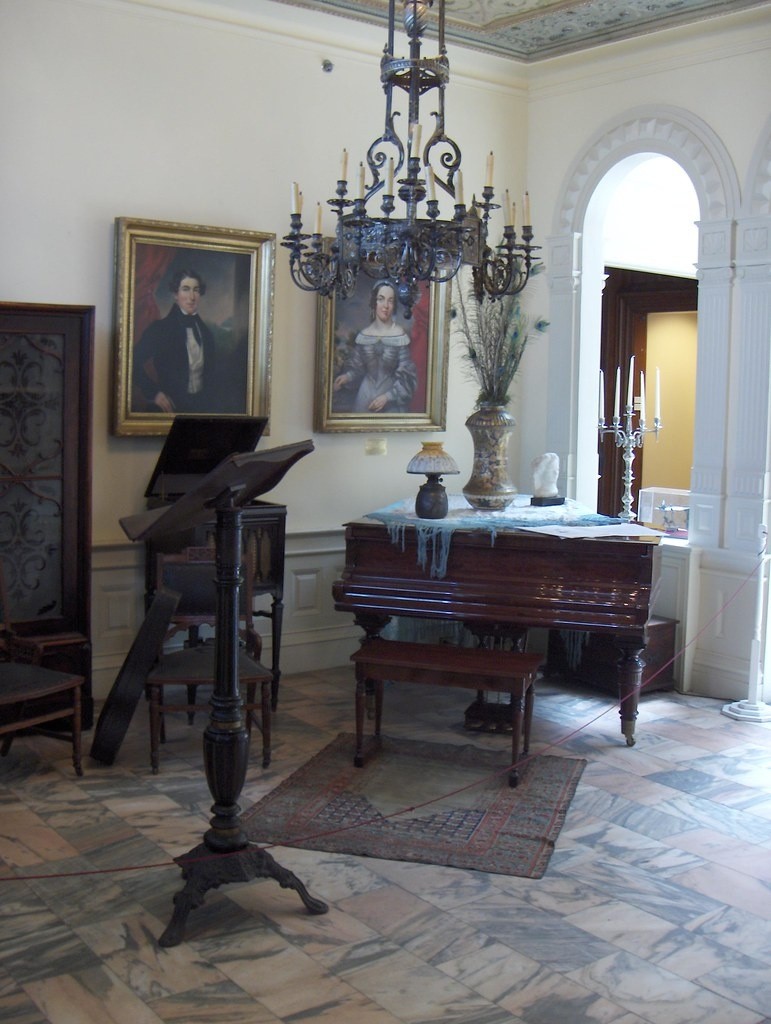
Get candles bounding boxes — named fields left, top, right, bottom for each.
left=627, top=356, right=636, bottom=406
left=613, top=366, right=621, bottom=417
left=600, top=369, right=605, bottom=419
left=655, top=366, right=660, bottom=418
left=640, top=370, right=646, bottom=420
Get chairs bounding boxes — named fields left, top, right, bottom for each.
left=146, top=540, right=274, bottom=770
left=0, top=557, right=85, bottom=777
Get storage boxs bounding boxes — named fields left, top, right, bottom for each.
left=638, top=487, right=690, bottom=539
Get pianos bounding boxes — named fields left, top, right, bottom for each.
left=333, top=516, right=652, bottom=748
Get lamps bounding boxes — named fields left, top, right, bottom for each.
left=279, top=0, right=544, bottom=321
left=405, top=441, right=461, bottom=519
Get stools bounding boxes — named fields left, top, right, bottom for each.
left=541, top=614, right=679, bottom=700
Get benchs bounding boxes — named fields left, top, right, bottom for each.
left=347, top=638, right=547, bottom=788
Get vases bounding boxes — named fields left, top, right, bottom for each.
left=462, top=403, right=521, bottom=513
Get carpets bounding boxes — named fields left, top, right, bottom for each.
left=237, top=732, right=588, bottom=881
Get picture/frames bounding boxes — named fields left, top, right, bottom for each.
left=111, top=217, right=278, bottom=437
left=313, top=236, right=453, bottom=433
